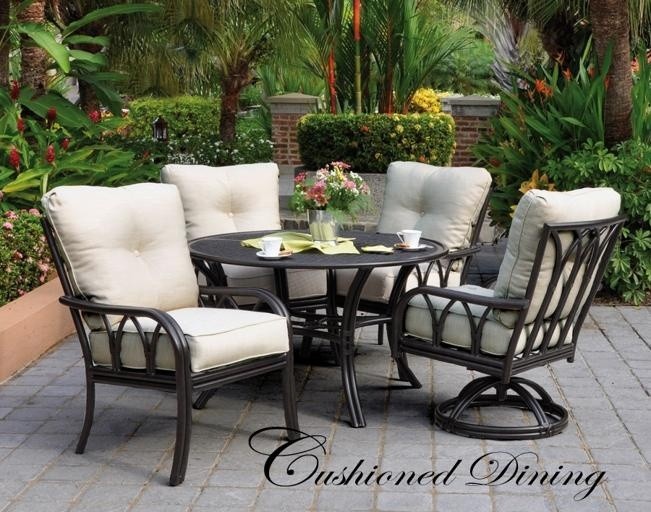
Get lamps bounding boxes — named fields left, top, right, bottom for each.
left=150, top=114, right=169, bottom=141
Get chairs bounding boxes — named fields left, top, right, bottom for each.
left=391, top=187, right=631, bottom=440
left=337, top=160, right=495, bottom=381
left=159, top=162, right=327, bottom=349
left=40, top=182, right=300, bottom=485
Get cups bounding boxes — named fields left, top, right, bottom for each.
left=396, top=229, right=422, bottom=247
left=258, top=237, right=283, bottom=257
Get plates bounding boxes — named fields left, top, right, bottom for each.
left=394, top=242, right=428, bottom=250
left=255, top=250, right=291, bottom=261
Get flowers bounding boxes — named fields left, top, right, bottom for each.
left=288, top=159, right=379, bottom=227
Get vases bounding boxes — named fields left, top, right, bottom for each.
left=307, top=209, right=336, bottom=242
